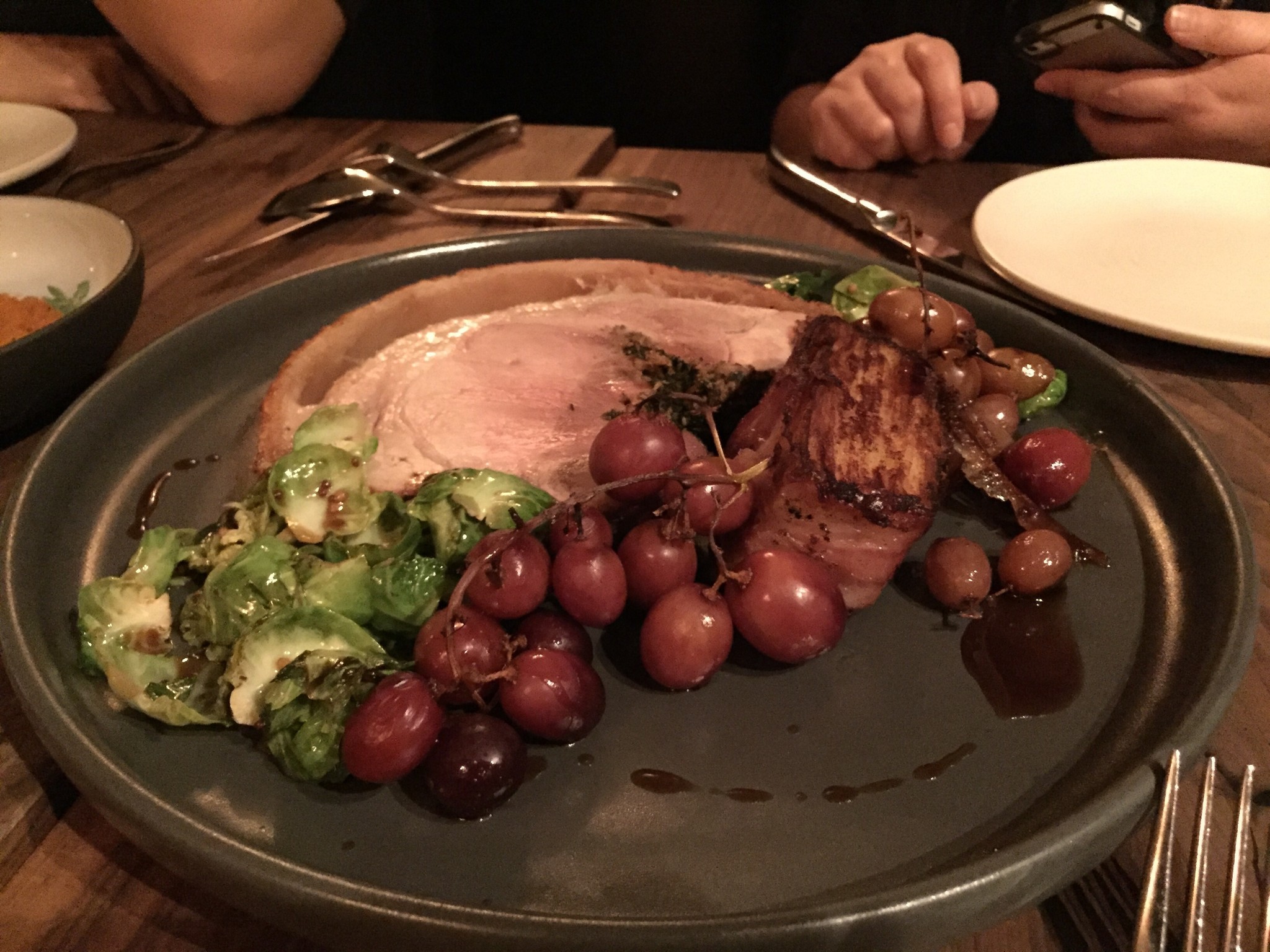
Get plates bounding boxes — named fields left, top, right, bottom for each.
left=0, top=98, right=78, bottom=188
left=0, top=211, right=1250, bottom=952
left=969, top=158, right=1270, bottom=357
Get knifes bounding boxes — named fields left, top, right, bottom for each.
left=769, top=145, right=1066, bottom=317
left=200, top=111, right=524, bottom=263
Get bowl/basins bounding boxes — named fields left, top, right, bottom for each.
left=2, top=195, right=144, bottom=453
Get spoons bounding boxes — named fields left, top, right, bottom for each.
left=255, top=165, right=673, bottom=234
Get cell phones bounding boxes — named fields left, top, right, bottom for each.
left=1011, top=0, right=1208, bottom=89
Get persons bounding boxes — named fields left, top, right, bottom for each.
left=0, top=2, right=394, bottom=121
left=727, top=0, right=1270, bottom=171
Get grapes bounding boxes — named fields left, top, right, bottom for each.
left=868, top=209, right=1091, bottom=510
left=342, top=413, right=847, bottom=818
left=931, top=529, right=988, bottom=620
left=993, top=529, right=1072, bottom=597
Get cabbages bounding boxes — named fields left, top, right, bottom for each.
left=74, top=448, right=561, bottom=781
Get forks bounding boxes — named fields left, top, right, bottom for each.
left=343, top=140, right=680, bottom=201
left=1129, top=746, right=1269, bottom=952
